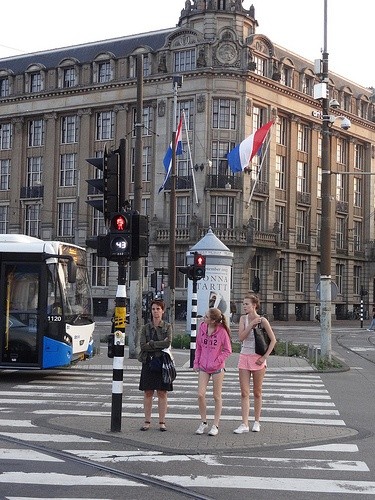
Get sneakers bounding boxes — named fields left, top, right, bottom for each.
left=209, top=425, right=218, bottom=435
left=252, top=422, right=261, bottom=431
left=233, top=424, right=249, bottom=433
left=196, top=421, right=208, bottom=434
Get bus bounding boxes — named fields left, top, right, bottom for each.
left=0, top=233, right=96, bottom=372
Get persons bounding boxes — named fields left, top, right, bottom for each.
left=139, top=300, right=173, bottom=431
left=233, top=296, right=277, bottom=433
left=193, top=308, right=232, bottom=436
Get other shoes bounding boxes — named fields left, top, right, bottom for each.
left=159, top=422, right=167, bottom=431
left=140, top=421, right=150, bottom=430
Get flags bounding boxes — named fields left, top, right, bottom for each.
left=227, top=121, right=274, bottom=172
left=157, top=118, right=184, bottom=192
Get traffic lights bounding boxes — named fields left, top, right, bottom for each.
left=129, top=214, right=150, bottom=261
left=109, top=213, right=129, bottom=257
left=179, top=266, right=194, bottom=280
left=85, top=147, right=120, bottom=216
left=85, top=235, right=110, bottom=258
left=151, top=273, right=156, bottom=288
left=195, top=254, right=206, bottom=280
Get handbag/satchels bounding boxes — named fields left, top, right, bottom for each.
left=254, top=315, right=275, bottom=356
left=161, top=352, right=176, bottom=384
